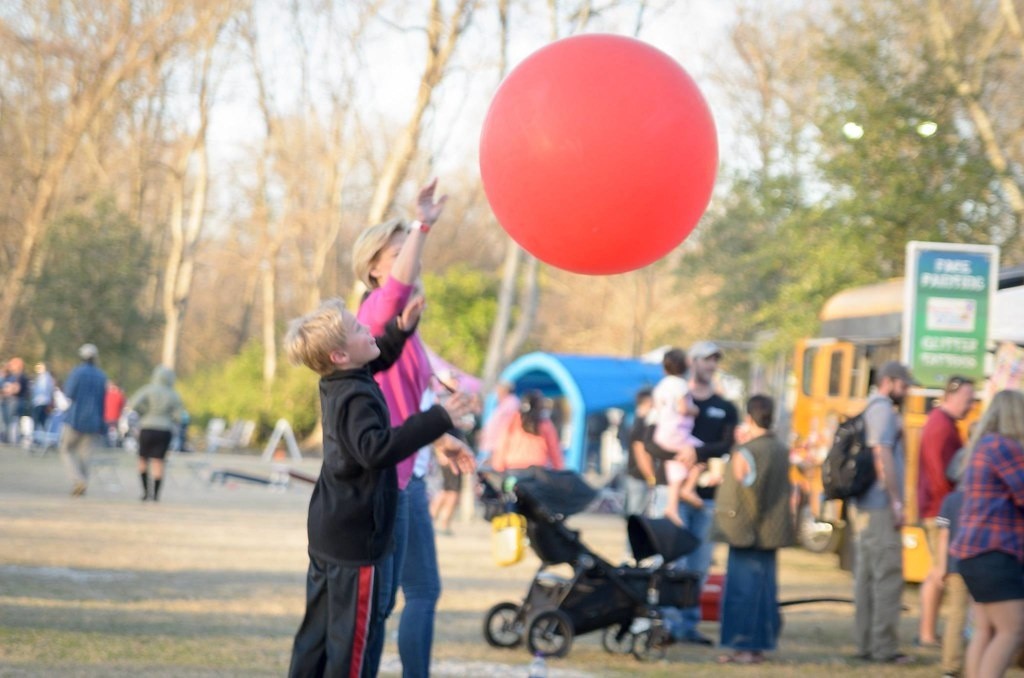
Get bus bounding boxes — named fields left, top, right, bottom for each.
left=783, top=277, right=987, bottom=584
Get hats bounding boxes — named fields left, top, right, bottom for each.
left=876, top=361, right=920, bottom=388
left=690, top=343, right=724, bottom=359
left=80, top=343, right=98, bottom=360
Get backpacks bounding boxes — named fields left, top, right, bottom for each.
left=821, top=399, right=893, bottom=497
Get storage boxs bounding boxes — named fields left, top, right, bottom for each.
left=702, top=578, right=724, bottom=620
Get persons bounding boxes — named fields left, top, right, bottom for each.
left=285, top=291, right=473, bottom=678
left=919, top=375, right=1024, bottom=678
left=352, top=176, right=441, bottom=678
left=0, top=342, right=189, bottom=501
left=434, top=341, right=794, bottom=664
left=845, top=362, right=923, bottom=666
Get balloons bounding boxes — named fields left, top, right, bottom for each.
left=480, top=34, right=715, bottom=275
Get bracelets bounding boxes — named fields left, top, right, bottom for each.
left=411, top=219, right=431, bottom=233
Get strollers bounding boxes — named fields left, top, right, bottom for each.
left=472, top=465, right=704, bottom=663
